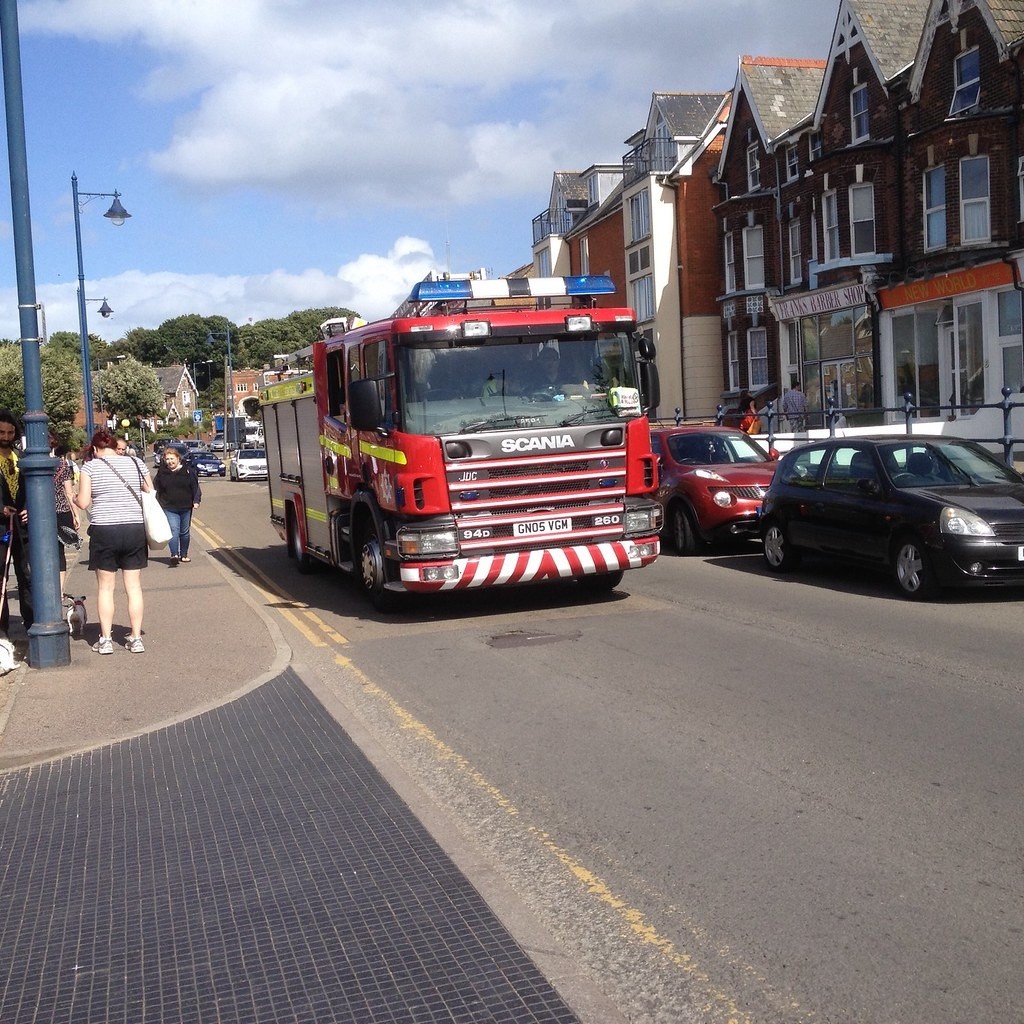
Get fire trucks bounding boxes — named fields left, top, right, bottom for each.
left=242, top=269, right=666, bottom=617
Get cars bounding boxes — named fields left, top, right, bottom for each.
left=760, top=434, right=1024, bottom=603
left=128, top=443, right=146, bottom=464
left=152, top=417, right=266, bottom=469
left=649, top=426, right=781, bottom=556
left=230, top=448, right=269, bottom=482
left=182, top=451, right=226, bottom=477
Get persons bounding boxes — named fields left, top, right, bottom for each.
left=71, top=431, right=153, bottom=656
left=49, top=441, right=80, bottom=486
left=527, top=347, right=579, bottom=400
left=828, top=379, right=849, bottom=426
left=0, top=412, right=35, bottom=641
left=783, top=381, right=810, bottom=433
left=127, top=440, right=139, bottom=453
left=152, top=447, right=202, bottom=565
left=48, top=431, right=81, bottom=603
left=82, top=446, right=95, bottom=466
left=739, top=390, right=760, bottom=434
left=114, top=438, right=131, bottom=457
left=858, top=383, right=873, bottom=408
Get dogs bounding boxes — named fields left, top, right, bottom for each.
left=66, top=595, right=86, bottom=636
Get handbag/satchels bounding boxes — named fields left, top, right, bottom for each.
left=130, top=456, right=174, bottom=550
left=746, top=418, right=762, bottom=435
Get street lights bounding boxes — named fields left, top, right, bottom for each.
left=121, top=418, right=130, bottom=446
left=98, top=354, right=127, bottom=431
left=205, top=323, right=241, bottom=450
left=76, top=286, right=115, bottom=442
left=193, top=359, right=214, bottom=388
left=70, top=169, right=134, bottom=442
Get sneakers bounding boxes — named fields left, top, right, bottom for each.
left=92, top=631, right=113, bottom=654
left=124, top=632, right=145, bottom=653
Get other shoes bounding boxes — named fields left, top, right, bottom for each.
left=0, top=630, right=11, bottom=642
left=170, top=557, right=179, bottom=567
left=181, top=556, right=191, bottom=562
